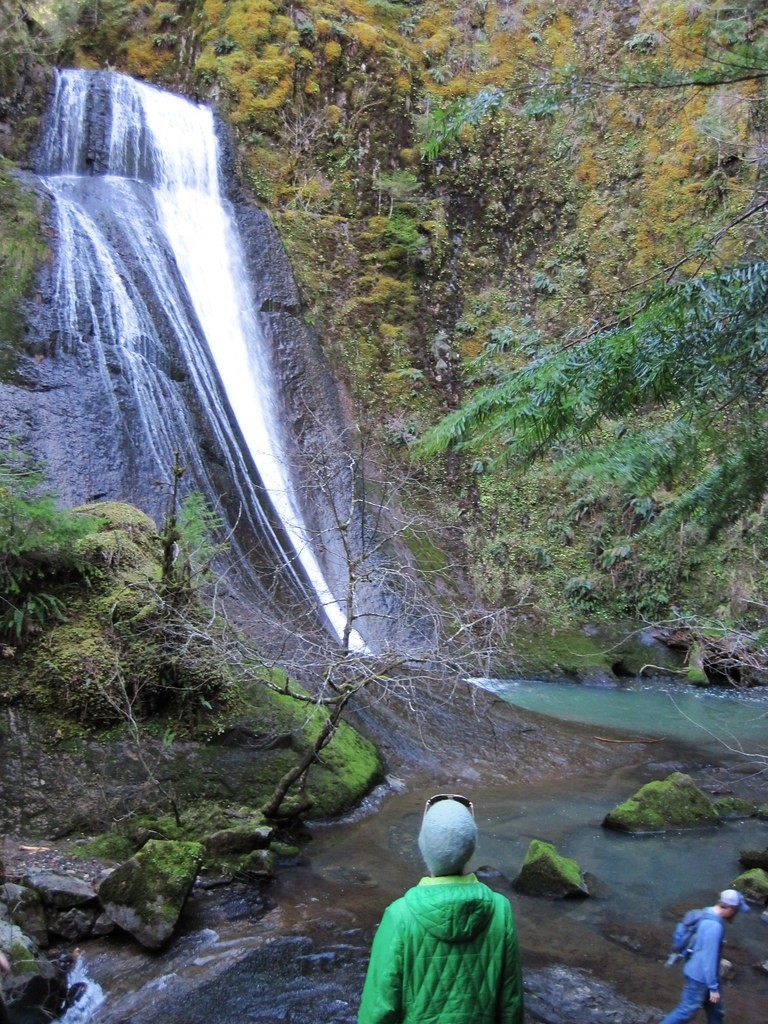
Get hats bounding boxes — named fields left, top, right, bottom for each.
left=718, top=888, right=752, bottom=914
left=417, top=800, right=478, bottom=877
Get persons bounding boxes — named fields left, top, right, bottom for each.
left=659, top=890, right=750, bottom=1024
left=357, top=793, right=523, bottom=1024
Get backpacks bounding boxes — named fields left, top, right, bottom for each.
left=672, top=906, right=727, bottom=958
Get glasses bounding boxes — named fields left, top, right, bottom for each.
left=422, top=792, right=476, bottom=822
